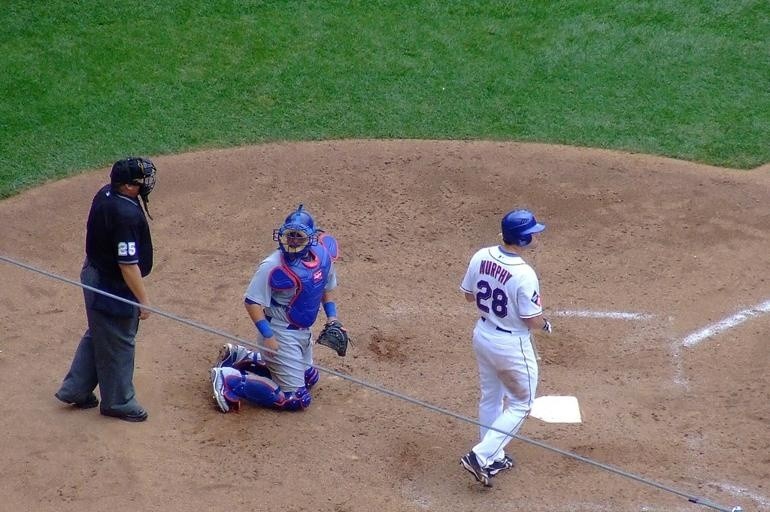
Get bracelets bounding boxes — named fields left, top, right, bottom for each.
left=322, top=302, right=337, bottom=317
left=255, top=319, right=275, bottom=339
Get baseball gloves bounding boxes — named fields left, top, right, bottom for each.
left=315, top=324, right=347, bottom=356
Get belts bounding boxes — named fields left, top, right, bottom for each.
left=481, top=317, right=511, bottom=333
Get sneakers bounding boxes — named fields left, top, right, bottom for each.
left=55, top=392, right=99, bottom=409
left=210, top=342, right=232, bottom=413
left=100, top=401, right=148, bottom=422
left=459, top=451, right=513, bottom=488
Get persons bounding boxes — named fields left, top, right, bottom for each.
left=53, top=158, right=156, bottom=422
left=208, top=204, right=349, bottom=414
left=460, top=209, right=552, bottom=490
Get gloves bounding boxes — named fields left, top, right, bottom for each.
left=543, top=317, right=552, bottom=336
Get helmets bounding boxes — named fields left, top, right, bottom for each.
left=110, top=156, right=156, bottom=192
left=502, top=209, right=546, bottom=246
left=279, top=205, right=316, bottom=259
